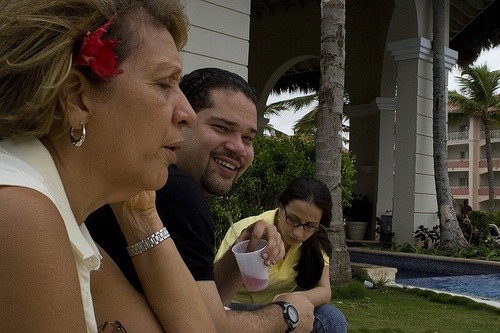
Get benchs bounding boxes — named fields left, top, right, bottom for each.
left=351, top=261, right=398, bottom=285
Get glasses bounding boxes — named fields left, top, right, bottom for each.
left=283, top=204, right=320, bottom=233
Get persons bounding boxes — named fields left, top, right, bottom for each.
left=213, top=177, right=348, bottom=333
left=0, top=0, right=218, bottom=333
left=85, top=67, right=316, bottom=333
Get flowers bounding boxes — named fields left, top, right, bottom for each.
left=74, top=14, right=125, bottom=83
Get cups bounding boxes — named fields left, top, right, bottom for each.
left=232, top=239, right=269, bottom=292
left=363, top=280, right=374, bottom=288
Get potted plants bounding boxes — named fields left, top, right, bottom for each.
left=342, top=193, right=374, bottom=240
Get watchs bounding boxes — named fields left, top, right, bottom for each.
left=126, top=227, right=170, bottom=257
left=272, top=301, right=300, bottom=333
